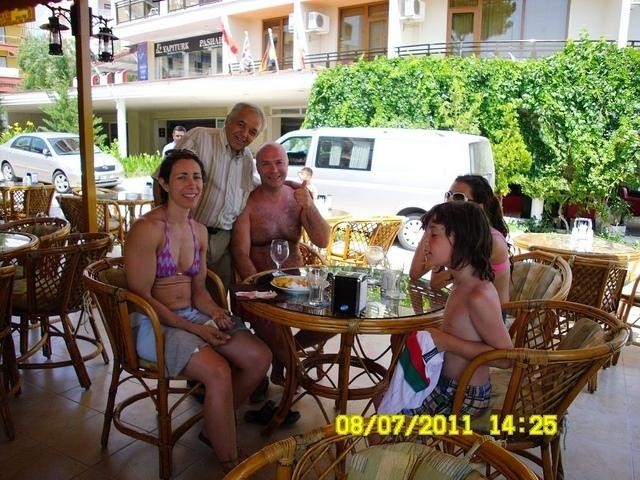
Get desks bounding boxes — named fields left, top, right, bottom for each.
left=514, top=232, right=637, bottom=257
left=236, top=266, right=450, bottom=480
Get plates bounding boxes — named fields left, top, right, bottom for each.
left=268, top=277, right=329, bottom=295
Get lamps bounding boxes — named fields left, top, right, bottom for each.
left=38, top=6, right=70, bottom=57
left=90, top=15, right=120, bottom=64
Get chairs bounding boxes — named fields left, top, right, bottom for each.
left=84, top=256, right=234, bottom=480
left=2, top=185, right=154, bottom=441
left=449, top=245, right=639, bottom=477
left=299, top=214, right=404, bottom=266
left=225, top=416, right=538, bottom=478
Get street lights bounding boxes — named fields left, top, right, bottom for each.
left=39, top=0, right=120, bottom=233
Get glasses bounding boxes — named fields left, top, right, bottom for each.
left=441, top=188, right=474, bottom=205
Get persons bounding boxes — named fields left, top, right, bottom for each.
left=151, top=102, right=267, bottom=305
left=161, top=124, right=187, bottom=161
left=410, top=176, right=515, bottom=324
left=401, top=201, right=515, bottom=423
left=231, top=142, right=338, bottom=393
left=123, top=150, right=272, bottom=474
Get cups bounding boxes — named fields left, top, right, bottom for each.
left=308, top=267, right=324, bottom=306
left=381, top=262, right=404, bottom=298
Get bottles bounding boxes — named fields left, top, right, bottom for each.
left=570, top=222, right=593, bottom=242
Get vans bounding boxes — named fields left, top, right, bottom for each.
left=252, top=127, right=496, bottom=251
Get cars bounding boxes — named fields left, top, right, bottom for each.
left=0, top=131, right=124, bottom=195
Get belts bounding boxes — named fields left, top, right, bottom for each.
left=200, top=224, right=232, bottom=235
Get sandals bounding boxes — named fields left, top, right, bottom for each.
left=245, top=402, right=301, bottom=427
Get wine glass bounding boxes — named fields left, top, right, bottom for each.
left=365, top=246, right=383, bottom=285
left=270, top=239, right=290, bottom=277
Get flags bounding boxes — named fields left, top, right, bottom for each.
left=259, top=33, right=276, bottom=72
left=221, top=23, right=238, bottom=66
left=239, top=33, right=253, bottom=75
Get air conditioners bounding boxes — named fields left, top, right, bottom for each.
left=307, top=11, right=332, bottom=33
left=339, top=20, right=353, bottom=41
left=404, top=0, right=425, bottom=23
